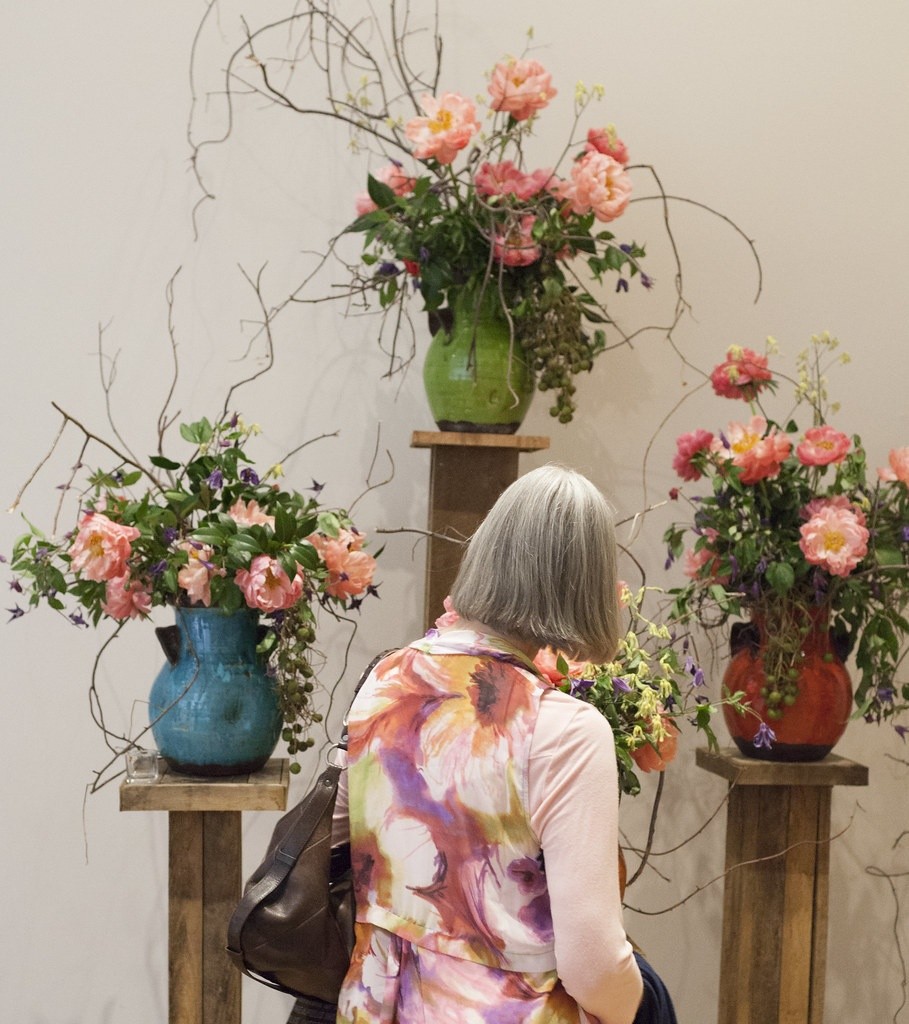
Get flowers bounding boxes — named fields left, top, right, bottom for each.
left=0, top=412, right=376, bottom=775
left=434, top=332, right=909, bottom=799
left=327, top=28, right=655, bottom=423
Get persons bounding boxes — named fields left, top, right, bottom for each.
left=228, top=465, right=677, bottom=1024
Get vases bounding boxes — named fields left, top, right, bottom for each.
left=419, top=283, right=538, bottom=432
left=722, top=597, right=853, bottom=763
left=148, top=604, right=286, bottom=779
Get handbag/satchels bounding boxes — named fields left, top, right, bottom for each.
left=227, top=645, right=401, bottom=1003
